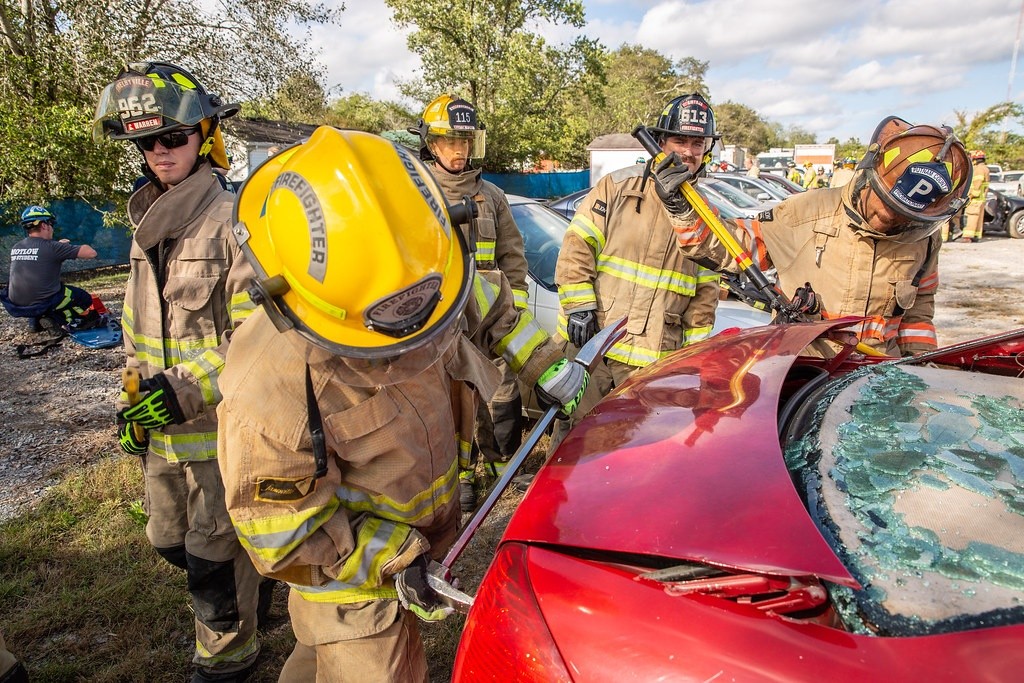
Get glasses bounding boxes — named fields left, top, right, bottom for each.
left=136, top=127, right=199, bottom=151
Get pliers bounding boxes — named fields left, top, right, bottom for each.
left=632, top=125, right=864, bottom=365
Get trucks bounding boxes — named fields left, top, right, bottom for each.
left=754, top=144, right=836, bottom=184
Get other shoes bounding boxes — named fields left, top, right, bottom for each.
left=191, top=661, right=258, bottom=683
left=460, top=479, right=477, bottom=512
left=509, top=474, right=536, bottom=493
left=257, top=578, right=277, bottom=624
left=954, top=237, right=973, bottom=243
left=27, top=319, right=43, bottom=333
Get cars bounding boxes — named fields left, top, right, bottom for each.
left=504, top=163, right=806, bottom=420
left=450, top=314, right=1024, bottom=683
left=960, top=164, right=1024, bottom=238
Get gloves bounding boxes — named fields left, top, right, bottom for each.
left=395, top=552, right=460, bottom=622
left=534, top=358, right=591, bottom=421
left=117, top=422, right=148, bottom=456
left=567, top=310, right=601, bottom=348
left=655, top=151, right=693, bottom=214
left=116, top=378, right=174, bottom=429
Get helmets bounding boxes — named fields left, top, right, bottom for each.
left=842, top=157, right=858, bottom=165
left=109, top=62, right=242, bottom=140
left=803, top=161, right=813, bottom=167
left=789, top=162, right=796, bottom=167
left=971, top=150, right=987, bottom=159
left=646, top=92, right=723, bottom=139
left=818, top=166, right=825, bottom=171
left=231, top=125, right=479, bottom=359
left=856, top=116, right=973, bottom=221
left=21, top=206, right=56, bottom=228
left=833, top=159, right=842, bottom=165
left=408, top=95, right=477, bottom=139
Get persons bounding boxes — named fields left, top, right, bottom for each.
left=787, top=161, right=828, bottom=189
left=216, top=126, right=591, bottom=683
left=407, top=94, right=528, bottom=512
left=744, top=158, right=761, bottom=178
left=831, top=155, right=858, bottom=188
left=941, top=209, right=963, bottom=243
left=546, top=91, right=721, bottom=459
left=92, top=60, right=278, bottom=683
left=654, top=114, right=974, bottom=360
left=0, top=206, right=97, bottom=332
left=705, top=159, right=720, bottom=173
left=956, top=150, right=991, bottom=242
